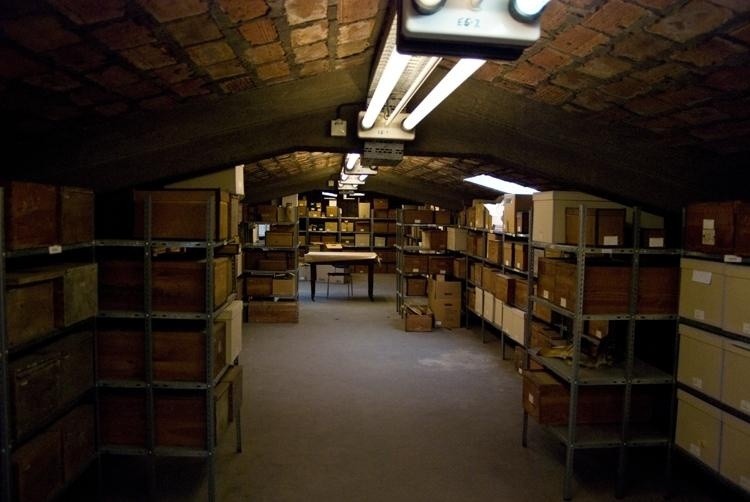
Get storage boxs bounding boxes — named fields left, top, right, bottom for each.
left=217, top=301, right=244, bottom=366
left=248, top=300, right=300, bottom=324
left=246, top=274, right=272, bottom=296
left=671, top=387, right=749, bottom=492
left=402, top=276, right=427, bottom=296
left=475, top=288, right=483, bottom=316
left=148, top=254, right=229, bottom=311
left=468, top=261, right=499, bottom=294
left=220, top=366, right=244, bottom=423
left=502, top=305, right=530, bottom=346
left=95, top=190, right=147, bottom=239
left=405, top=302, right=435, bottom=334
left=264, top=227, right=297, bottom=247
left=467, top=286, right=475, bottom=309
left=397, top=202, right=487, bottom=258
left=3, top=418, right=60, bottom=498
left=59, top=330, right=95, bottom=409
left=516, top=211, right=529, bottom=234
left=529, top=192, right=617, bottom=250
left=96, top=327, right=149, bottom=380
left=92, top=387, right=147, bottom=448
left=61, top=413, right=92, bottom=480
left=556, top=259, right=680, bottom=314
left=148, top=187, right=229, bottom=241
left=58, top=186, right=94, bottom=245
left=280, top=193, right=396, bottom=248
left=680, top=256, right=748, bottom=338
left=0, top=270, right=60, bottom=351
left=428, top=257, right=453, bottom=275
left=60, top=260, right=98, bottom=329
left=533, top=255, right=568, bottom=304
left=503, top=241, right=514, bottom=267
left=566, top=205, right=627, bottom=247
left=496, top=273, right=527, bottom=304
left=157, top=164, right=245, bottom=195
left=675, top=322, right=749, bottom=418
left=684, top=199, right=750, bottom=257
left=453, top=257, right=467, bottom=279
left=484, top=290, right=495, bottom=322
left=2, top=179, right=58, bottom=250
left=494, top=298, right=503, bottom=327
left=429, top=279, right=463, bottom=330
left=150, top=322, right=228, bottom=383
left=98, top=258, right=148, bottom=313
left=244, top=248, right=299, bottom=270
left=516, top=278, right=537, bottom=313
left=503, top=193, right=532, bottom=232
left=489, top=238, right=502, bottom=263
left=229, top=196, right=239, bottom=238
left=524, top=368, right=641, bottom=428
left=400, top=253, right=427, bottom=273
left=148, top=384, right=231, bottom=448
left=514, top=242, right=529, bottom=270
left=5, top=343, right=60, bottom=445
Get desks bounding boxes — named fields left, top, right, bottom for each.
left=305, top=251, right=378, bottom=301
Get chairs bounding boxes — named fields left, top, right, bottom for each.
left=326, top=261, right=355, bottom=300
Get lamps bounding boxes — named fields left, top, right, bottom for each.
left=356, top=9, right=523, bottom=141
left=342, top=150, right=379, bottom=175
